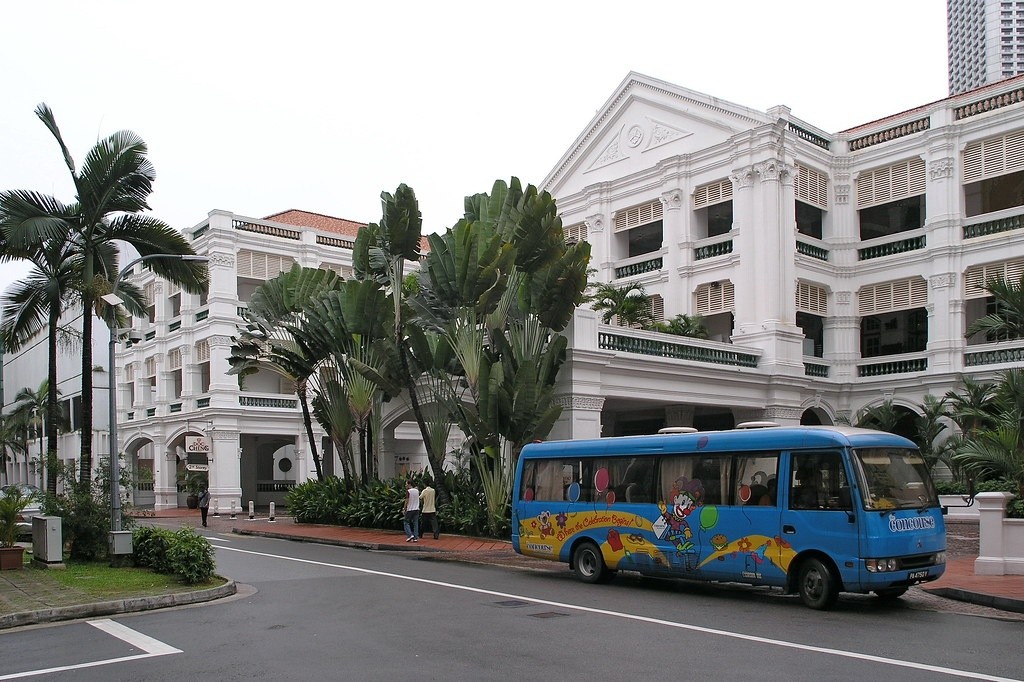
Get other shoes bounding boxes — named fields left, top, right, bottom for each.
left=413, top=539, right=417, bottom=542
left=417, top=535, right=422, bottom=538
left=434, top=531, right=439, bottom=540
left=202, top=523, right=207, bottom=526
left=406, top=535, right=414, bottom=542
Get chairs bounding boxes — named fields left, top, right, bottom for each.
left=745, top=484, right=768, bottom=506
left=625, top=483, right=643, bottom=503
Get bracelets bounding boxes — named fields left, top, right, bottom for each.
left=403, top=508, right=405, bottom=510
left=206, top=504, right=208, bottom=506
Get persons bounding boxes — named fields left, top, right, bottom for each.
left=608, top=478, right=778, bottom=507
left=197, top=485, right=212, bottom=528
left=402, top=480, right=439, bottom=542
left=843, top=466, right=893, bottom=498
left=795, top=458, right=823, bottom=505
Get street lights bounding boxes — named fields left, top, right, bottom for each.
left=104, top=254, right=208, bottom=565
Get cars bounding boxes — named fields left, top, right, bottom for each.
left=0, top=483, right=75, bottom=537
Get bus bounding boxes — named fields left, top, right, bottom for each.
left=510, top=420, right=978, bottom=608
